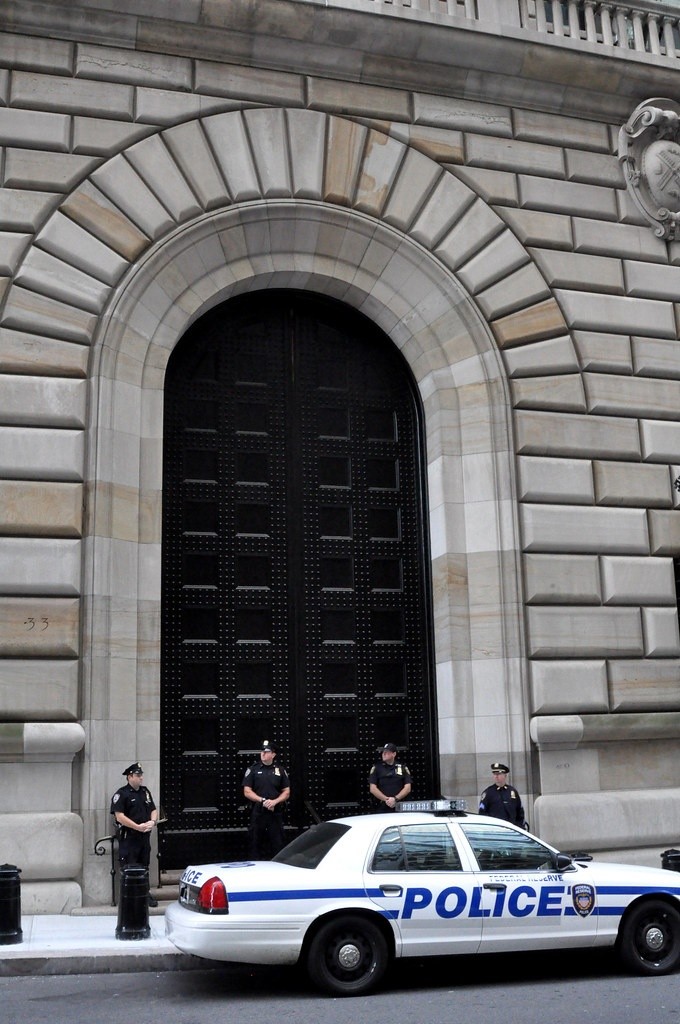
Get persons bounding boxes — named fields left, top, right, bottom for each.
left=108, top=763, right=163, bottom=906
left=240, top=737, right=292, bottom=862
left=368, top=742, right=411, bottom=813
left=478, top=763, right=526, bottom=831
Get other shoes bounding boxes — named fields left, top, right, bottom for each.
left=148, top=896, right=158, bottom=907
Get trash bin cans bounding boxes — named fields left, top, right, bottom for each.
left=660, top=848, right=680, bottom=873
left=115, top=864, right=151, bottom=941
left=0, top=863, right=23, bottom=946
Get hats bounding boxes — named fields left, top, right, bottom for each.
left=260, top=740, right=276, bottom=752
left=491, top=762, right=509, bottom=774
left=380, top=743, right=398, bottom=754
left=122, top=762, right=143, bottom=775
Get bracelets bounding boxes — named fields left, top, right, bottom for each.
left=151, top=820, right=156, bottom=827
left=260, top=797, right=265, bottom=804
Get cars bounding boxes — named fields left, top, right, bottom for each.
left=165, top=799, right=680, bottom=997
